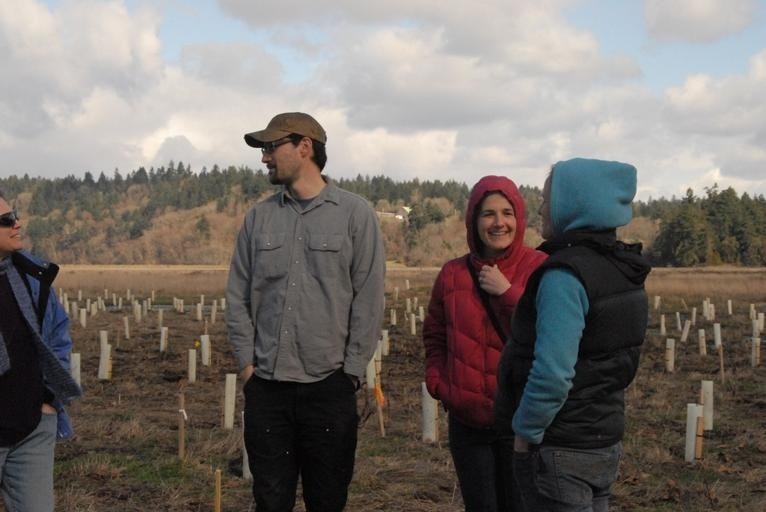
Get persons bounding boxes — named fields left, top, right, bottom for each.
left=220, top=112, right=386, bottom=512
left=0, top=192, right=84, bottom=512
left=489, top=156, right=653, bottom=511
left=421, top=173, right=552, bottom=511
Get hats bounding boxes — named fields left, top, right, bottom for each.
left=245, top=112, right=326, bottom=148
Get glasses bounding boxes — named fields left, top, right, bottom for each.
left=0, top=211, right=18, bottom=226
left=262, top=138, right=297, bottom=152
